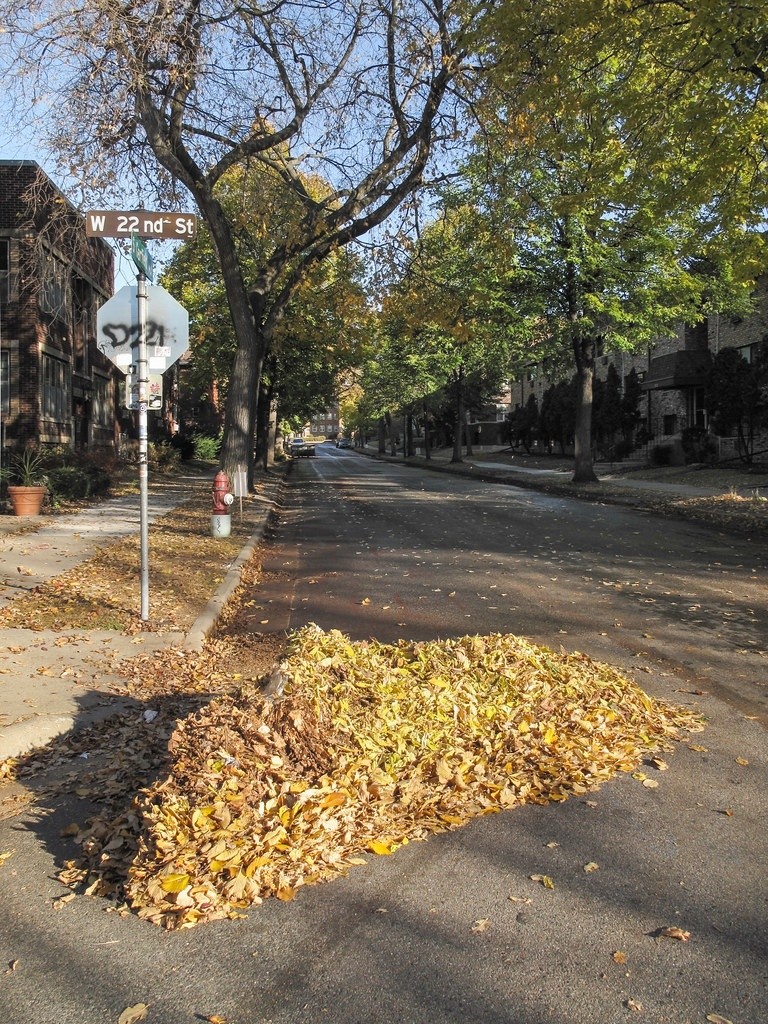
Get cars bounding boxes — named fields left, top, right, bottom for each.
left=291, top=438, right=305, bottom=449
left=338, top=438, right=351, bottom=449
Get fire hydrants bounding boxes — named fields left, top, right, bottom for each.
left=211, top=469, right=233, bottom=515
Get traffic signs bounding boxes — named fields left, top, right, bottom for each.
left=86, top=210, right=198, bottom=240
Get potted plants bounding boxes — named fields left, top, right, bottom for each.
left=0, top=445, right=49, bottom=516
